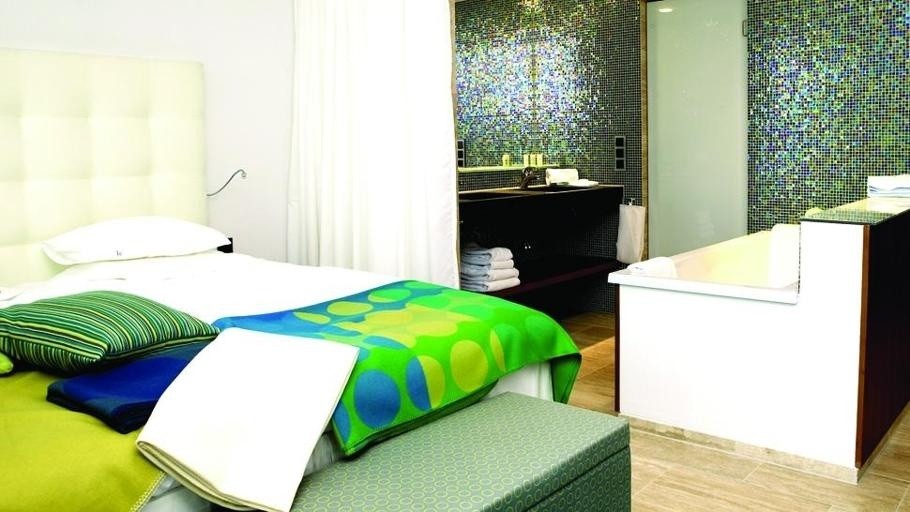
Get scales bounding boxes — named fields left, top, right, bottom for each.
left=608, top=228, right=799, bottom=305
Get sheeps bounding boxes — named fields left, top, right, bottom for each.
left=520, top=166, right=539, bottom=189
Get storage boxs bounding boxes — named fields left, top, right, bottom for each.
left=291, top=391, right=631, bottom=512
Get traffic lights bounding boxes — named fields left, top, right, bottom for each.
left=0, top=291, right=222, bottom=376
left=38, top=217, right=232, bottom=265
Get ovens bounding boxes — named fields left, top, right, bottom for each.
left=0, top=252, right=583, bottom=512
